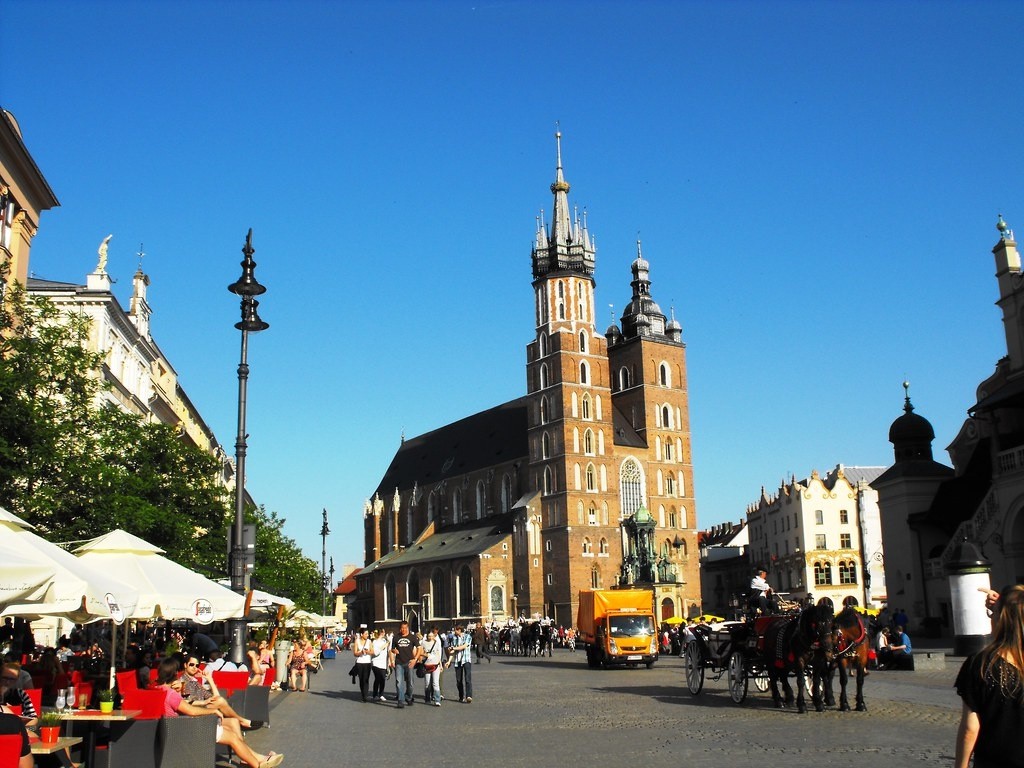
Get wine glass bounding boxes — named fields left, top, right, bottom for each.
left=66, top=687, right=76, bottom=712
left=55, top=689, right=66, bottom=715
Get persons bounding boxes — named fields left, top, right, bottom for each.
left=485, top=612, right=579, bottom=652
left=877, top=626, right=912, bottom=670
left=472, top=622, right=491, bottom=664
left=751, top=572, right=782, bottom=617
left=420, top=630, right=441, bottom=706
left=952, top=584, right=1024, bottom=768
left=354, top=628, right=374, bottom=703
left=977, top=587, right=999, bottom=609
left=432, top=624, right=445, bottom=700
left=445, top=624, right=473, bottom=703
left=656, top=617, right=719, bottom=658
left=0, top=619, right=353, bottom=768
left=371, top=628, right=390, bottom=701
left=391, top=621, right=423, bottom=707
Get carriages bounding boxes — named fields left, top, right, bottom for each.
left=487, top=619, right=555, bottom=658
left=686, top=602, right=869, bottom=713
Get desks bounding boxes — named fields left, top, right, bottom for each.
left=312, top=649, right=324, bottom=671
left=58, top=709, right=142, bottom=768
left=31, top=736, right=83, bottom=768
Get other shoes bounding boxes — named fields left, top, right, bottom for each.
left=474, top=661, right=480, bottom=664
left=488, top=657, right=491, bottom=663
left=259, top=753, right=285, bottom=768
left=300, top=687, right=307, bottom=692
left=405, top=697, right=413, bottom=706
left=291, top=687, right=299, bottom=692
left=467, top=696, right=473, bottom=703
left=459, top=698, right=465, bottom=702
left=240, top=720, right=266, bottom=732
left=397, top=700, right=403, bottom=708
left=361, top=695, right=398, bottom=702
left=424, top=695, right=445, bottom=707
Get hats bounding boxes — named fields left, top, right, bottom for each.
left=757, top=566, right=768, bottom=573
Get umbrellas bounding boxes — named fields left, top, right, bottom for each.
left=0, top=507, right=140, bottom=625
left=217, top=579, right=295, bottom=607
left=274, top=610, right=338, bottom=635
left=660, top=617, right=687, bottom=627
left=71, top=529, right=247, bottom=701
left=692, top=614, right=725, bottom=623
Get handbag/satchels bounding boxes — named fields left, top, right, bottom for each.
left=417, top=664, right=427, bottom=678
left=349, top=666, right=358, bottom=677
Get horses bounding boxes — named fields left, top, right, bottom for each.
left=762, top=603, right=870, bottom=714
left=487, top=619, right=553, bottom=657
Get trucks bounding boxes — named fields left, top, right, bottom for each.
left=575, top=589, right=663, bottom=670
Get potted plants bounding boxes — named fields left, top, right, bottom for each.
left=38, top=709, right=63, bottom=742
left=97, top=688, right=117, bottom=713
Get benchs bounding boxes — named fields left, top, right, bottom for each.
left=710, top=634, right=732, bottom=655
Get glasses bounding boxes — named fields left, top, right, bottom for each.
left=986, top=603, right=1002, bottom=619
left=187, top=662, right=199, bottom=668
left=0, top=676, right=19, bottom=684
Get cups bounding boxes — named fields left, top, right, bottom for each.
left=78, top=694, right=87, bottom=710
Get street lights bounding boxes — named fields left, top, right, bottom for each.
left=227, top=227, right=270, bottom=663
left=329, top=556, right=336, bottom=616
left=319, top=509, right=331, bottom=638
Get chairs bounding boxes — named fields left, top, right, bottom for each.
left=287, top=654, right=310, bottom=691
left=0, top=659, right=276, bottom=768
